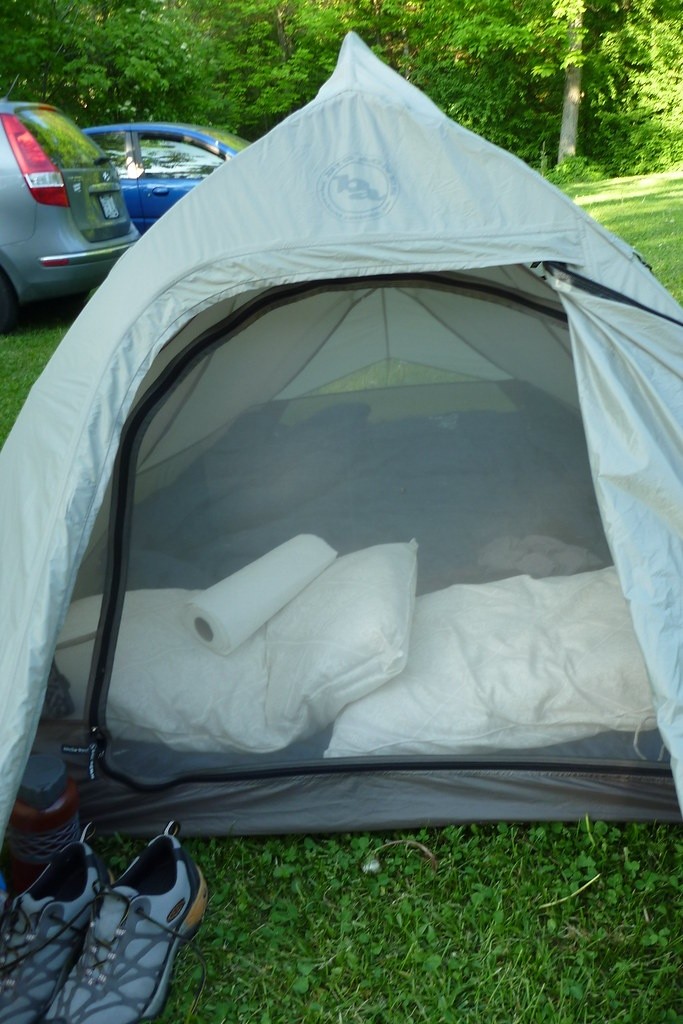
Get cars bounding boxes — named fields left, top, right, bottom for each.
left=80, top=122, right=254, bottom=238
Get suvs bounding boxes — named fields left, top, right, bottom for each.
left=0, top=98, right=141, bottom=331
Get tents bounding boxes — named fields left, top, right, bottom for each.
left=1, top=30, right=682, bottom=855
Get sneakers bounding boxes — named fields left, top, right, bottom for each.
left=0, top=823, right=115, bottom=1024
left=45, top=820, right=206, bottom=1024
